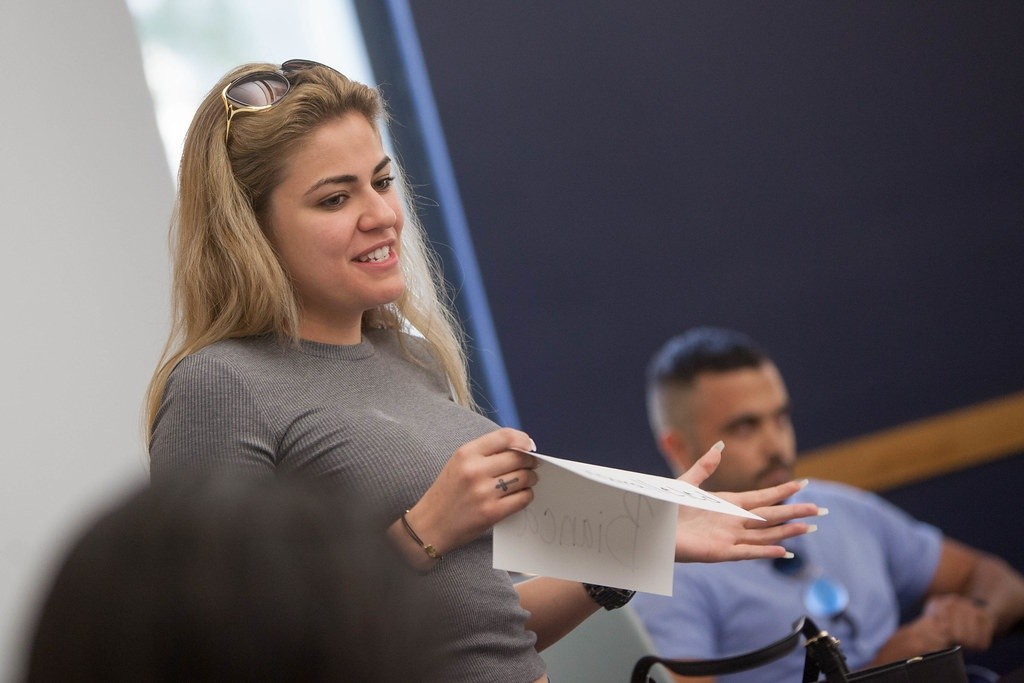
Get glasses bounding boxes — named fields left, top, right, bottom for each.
left=221, top=59, right=350, bottom=147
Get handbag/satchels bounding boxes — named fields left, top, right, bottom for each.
left=629, top=615, right=967, bottom=683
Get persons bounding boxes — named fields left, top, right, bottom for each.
left=145, top=58, right=828, bottom=683
left=627, top=327, right=1023, bottom=683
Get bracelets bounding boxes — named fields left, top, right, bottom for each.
left=402, top=510, right=444, bottom=561
left=583, top=582, right=636, bottom=612
